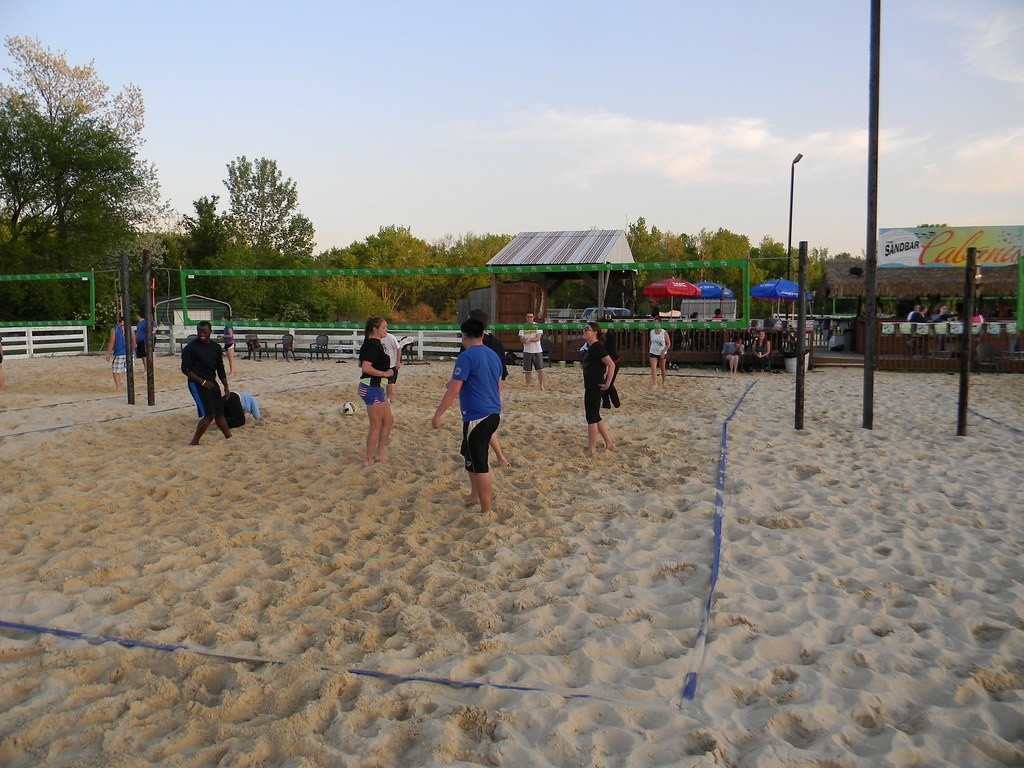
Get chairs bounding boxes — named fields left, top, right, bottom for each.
left=310, top=335, right=330, bottom=361
left=275, top=334, right=296, bottom=362
left=181, top=334, right=198, bottom=350
left=398, top=337, right=414, bottom=364
left=245, top=334, right=269, bottom=360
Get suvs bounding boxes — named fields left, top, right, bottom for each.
left=581, top=307, right=630, bottom=316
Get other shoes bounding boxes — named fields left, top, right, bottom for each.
left=769, top=371, right=779, bottom=375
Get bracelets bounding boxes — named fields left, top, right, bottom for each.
left=202, top=380, right=207, bottom=386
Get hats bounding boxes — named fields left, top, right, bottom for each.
left=650, top=298, right=656, bottom=303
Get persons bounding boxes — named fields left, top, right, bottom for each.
left=579, top=322, right=616, bottom=454
left=445, top=310, right=511, bottom=467
left=181, top=321, right=233, bottom=445
left=106, top=317, right=135, bottom=391
left=134, top=311, right=157, bottom=377
left=906, top=303, right=1012, bottom=358
left=518, top=310, right=544, bottom=390
left=219, top=314, right=234, bottom=375
left=358, top=316, right=395, bottom=467
left=221, top=390, right=261, bottom=429
left=648, top=318, right=671, bottom=388
left=721, top=338, right=745, bottom=374
left=431, top=318, right=503, bottom=512
left=751, top=330, right=771, bottom=373
left=648, top=299, right=727, bottom=322
left=600, top=329, right=621, bottom=409
left=380, top=333, right=401, bottom=403
left=0, top=335, right=5, bottom=388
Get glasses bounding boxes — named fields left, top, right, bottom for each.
left=527, top=316, right=532, bottom=318
left=585, top=327, right=594, bottom=331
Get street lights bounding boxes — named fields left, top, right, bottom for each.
left=786, top=154, right=804, bottom=320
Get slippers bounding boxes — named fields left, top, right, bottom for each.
left=336, top=360, right=347, bottom=363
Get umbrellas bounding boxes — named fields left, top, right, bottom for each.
left=641, top=276, right=701, bottom=319
left=684, top=280, right=733, bottom=318
left=748, top=276, right=813, bottom=318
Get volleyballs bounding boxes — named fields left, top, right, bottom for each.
left=343, top=403, right=356, bottom=414
